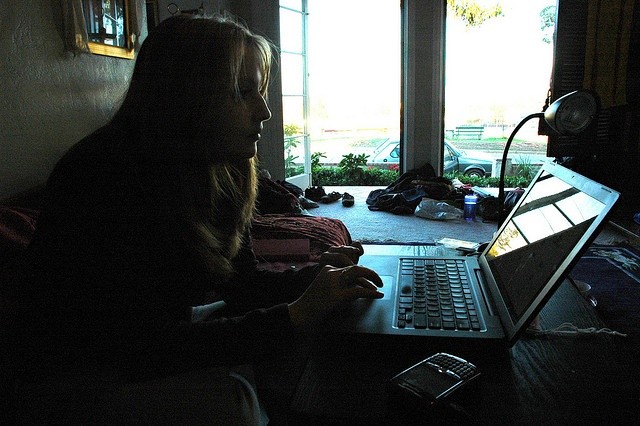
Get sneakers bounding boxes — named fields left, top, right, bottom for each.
left=305, top=185, right=326, bottom=202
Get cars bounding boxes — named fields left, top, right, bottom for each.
left=370, top=136, right=493, bottom=180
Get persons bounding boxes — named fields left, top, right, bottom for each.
left=1, top=15, right=384, bottom=425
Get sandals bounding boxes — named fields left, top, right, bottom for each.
left=321, top=192, right=341, bottom=203
left=342, top=193, right=354, bottom=206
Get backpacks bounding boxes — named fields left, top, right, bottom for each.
left=276, top=180, right=303, bottom=198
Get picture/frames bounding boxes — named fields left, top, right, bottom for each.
left=146, top=0, right=159, bottom=37
left=67, top=0, right=135, bottom=60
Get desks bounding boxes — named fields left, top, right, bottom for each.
left=287, top=242, right=640, bottom=426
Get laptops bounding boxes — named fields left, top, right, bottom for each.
left=357, top=161, right=622, bottom=349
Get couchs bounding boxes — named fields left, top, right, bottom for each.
left=0, top=185, right=354, bottom=426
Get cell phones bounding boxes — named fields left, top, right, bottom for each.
left=388, top=351, right=483, bottom=409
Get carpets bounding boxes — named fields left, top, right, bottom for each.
left=568, top=237, right=640, bottom=366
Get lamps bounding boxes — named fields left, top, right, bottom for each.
left=478, top=87, right=601, bottom=253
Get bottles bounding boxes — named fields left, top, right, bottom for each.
left=464, top=189, right=478, bottom=220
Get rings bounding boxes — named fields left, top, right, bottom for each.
left=340, top=269, right=350, bottom=283
